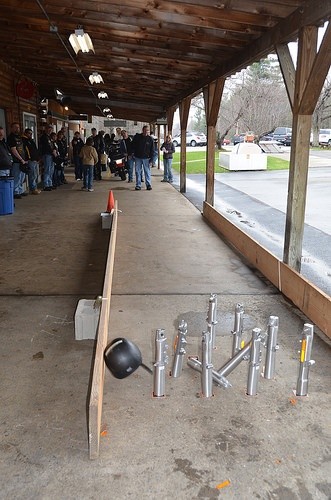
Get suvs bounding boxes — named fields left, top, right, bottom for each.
left=172, top=130, right=207, bottom=147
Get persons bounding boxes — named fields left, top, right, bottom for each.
left=98, top=127, right=123, bottom=171
left=72, top=131, right=83, bottom=181
left=7, top=122, right=29, bottom=199
left=78, top=137, right=98, bottom=192
left=39, top=125, right=68, bottom=191
left=120, top=130, right=144, bottom=182
left=21, top=128, right=41, bottom=195
left=149, top=132, right=158, bottom=168
left=89, top=128, right=105, bottom=180
left=160, top=135, right=175, bottom=183
left=0, top=127, right=13, bottom=176
left=130, top=125, right=155, bottom=190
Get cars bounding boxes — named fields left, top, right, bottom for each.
left=261, top=134, right=273, bottom=141
left=234, top=133, right=246, bottom=145
left=222, top=139, right=230, bottom=144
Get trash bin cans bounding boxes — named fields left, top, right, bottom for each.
left=0, top=176, right=15, bottom=214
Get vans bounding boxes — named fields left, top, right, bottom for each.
left=273, top=127, right=292, bottom=146
left=310, top=129, right=331, bottom=148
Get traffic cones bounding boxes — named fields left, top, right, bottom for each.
left=106, top=190, right=115, bottom=212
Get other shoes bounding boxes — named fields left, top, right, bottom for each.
left=14, top=194, right=22, bottom=200
left=80, top=186, right=86, bottom=191
left=167, top=180, right=173, bottom=183
left=88, top=188, right=94, bottom=192
left=20, top=192, right=27, bottom=196
left=29, top=188, right=41, bottom=195
left=43, top=186, right=57, bottom=191
left=147, top=186, right=152, bottom=190
left=135, top=186, right=141, bottom=190
left=161, top=179, right=168, bottom=182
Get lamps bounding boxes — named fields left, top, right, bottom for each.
left=39, top=87, right=65, bottom=114
left=107, top=114, right=113, bottom=118
left=69, top=25, right=95, bottom=55
left=89, top=72, right=105, bottom=85
left=98, top=91, right=109, bottom=99
left=103, top=108, right=111, bottom=113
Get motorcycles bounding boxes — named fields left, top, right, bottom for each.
left=107, top=142, right=130, bottom=181
